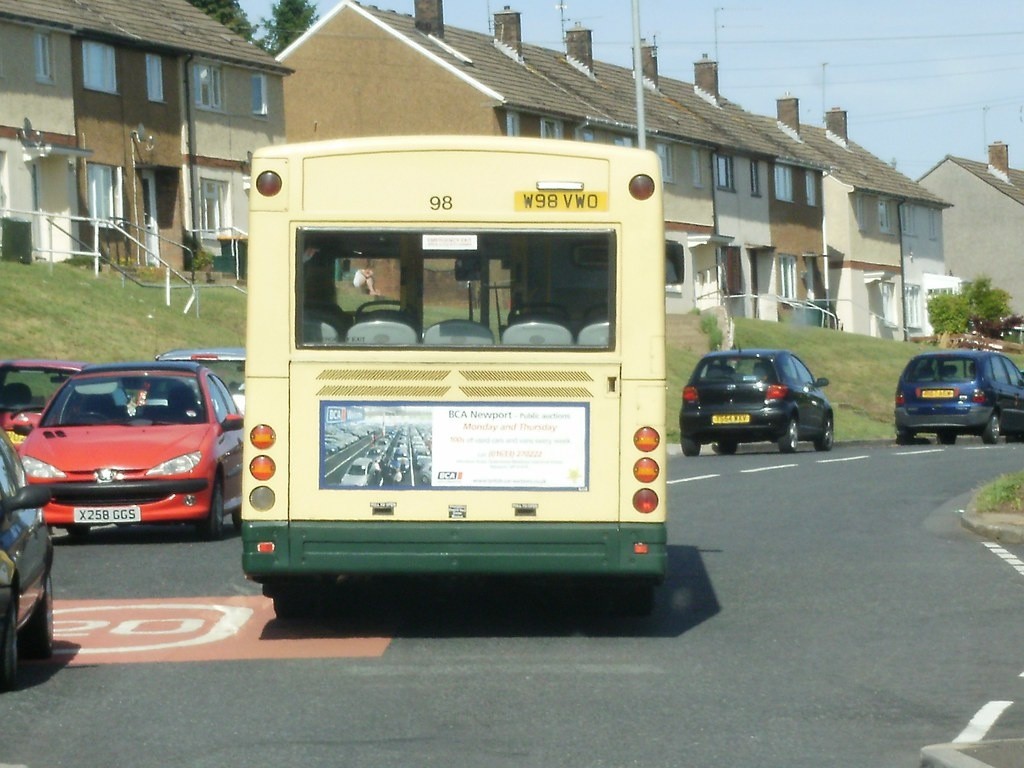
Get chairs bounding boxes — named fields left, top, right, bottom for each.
left=0, top=382, right=204, bottom=424
left=704, top=363, right=782, bottom=378
left=915, top=363, right=992, bottom=379
left=303, top=297, right=611, bottom=348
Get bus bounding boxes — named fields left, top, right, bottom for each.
left=235, top=130, right=688, bottom=624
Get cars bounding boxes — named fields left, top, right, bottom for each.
left=16, top=359, right=245, bottom=543
left=892, top=350, right=1024, bottom=445
left=136, top=348, right=246, bottom=436
left=0, top=359, right=130, bottom=451
left=678, top=344, right=837, bottom=458
left=0, top=425, right=58, bottom=693
